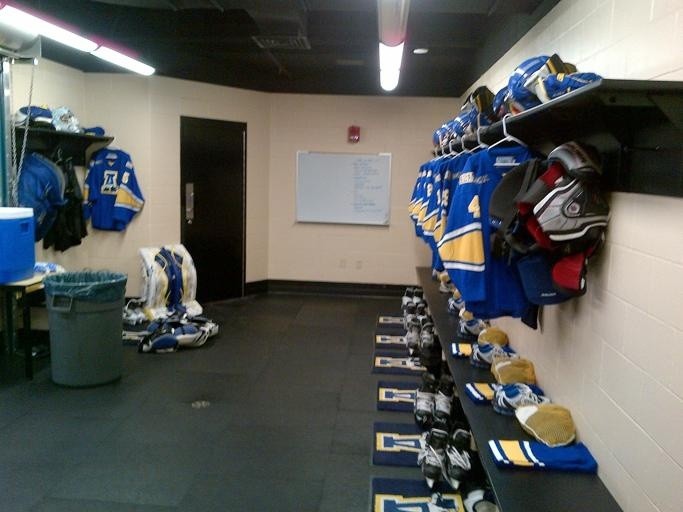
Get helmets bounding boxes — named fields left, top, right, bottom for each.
left=432, top=56, right=599, bottom=145
left=489, top=142, right=609, bottom=256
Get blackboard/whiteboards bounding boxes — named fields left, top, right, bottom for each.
left=296, top=150, right=392, bottom=226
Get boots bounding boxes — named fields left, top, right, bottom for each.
left=400, top=285, right=550, bottom=483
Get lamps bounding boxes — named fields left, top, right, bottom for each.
left=0, top=0, right=155, bottom=77
left=376, top=1, right=412, bottom=93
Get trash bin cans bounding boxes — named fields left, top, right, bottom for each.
left=42, top=271, right=127, bottom=386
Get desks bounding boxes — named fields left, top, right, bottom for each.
left=0, top=268, right=48, bottom=380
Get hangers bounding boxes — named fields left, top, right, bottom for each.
left=427, top=111, right=530, bottom=162
